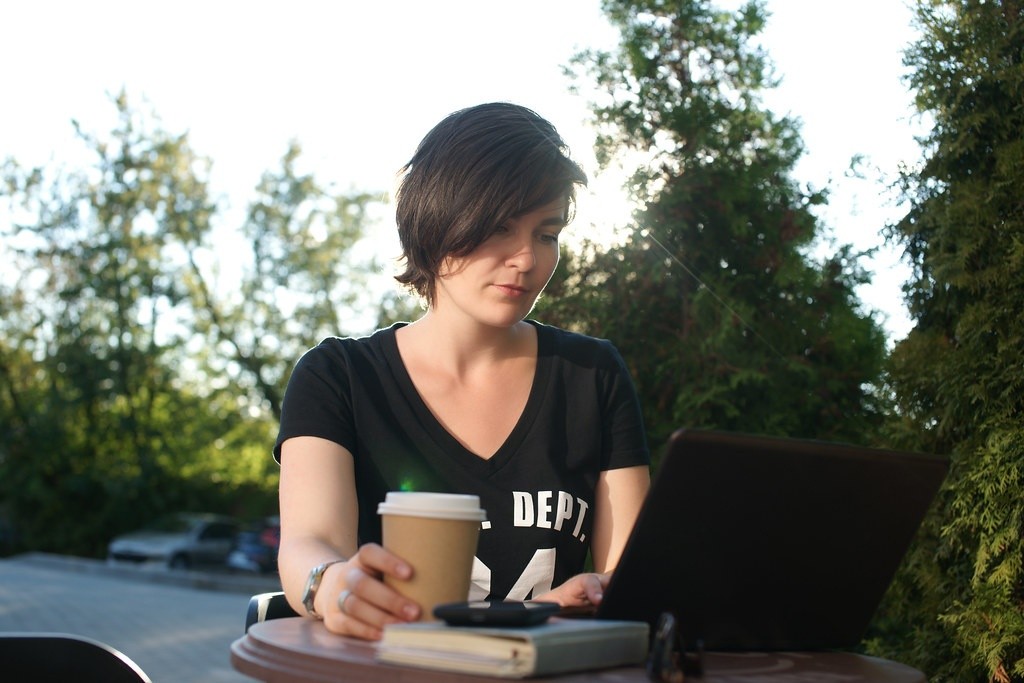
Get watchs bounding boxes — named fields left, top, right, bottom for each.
left=303, top=559, right=348, bottom=620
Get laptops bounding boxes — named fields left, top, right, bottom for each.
left=561, top=429, right=953, bottom=654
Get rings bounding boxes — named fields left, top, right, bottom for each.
left=339, top=590, right=351, bottom=613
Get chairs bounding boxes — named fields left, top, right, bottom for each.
left=1, top=635, right=152, bottom=683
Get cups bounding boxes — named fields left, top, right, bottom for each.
left=377, top=491, right=486, bottom=624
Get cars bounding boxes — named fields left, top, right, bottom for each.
left=108, top=511, right=242, bottom=570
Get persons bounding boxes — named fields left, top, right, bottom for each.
left=271, top=101, right=651, bottom=642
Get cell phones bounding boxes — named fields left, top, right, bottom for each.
left=434, top=601, right=558, bottom=627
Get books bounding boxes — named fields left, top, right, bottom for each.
left=373, top=619, right=649, bottom=678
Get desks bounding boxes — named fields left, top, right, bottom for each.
left=229, top=617, right=925, bottom=683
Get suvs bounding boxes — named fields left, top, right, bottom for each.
left=228, top=512, right=281, bottom=574
